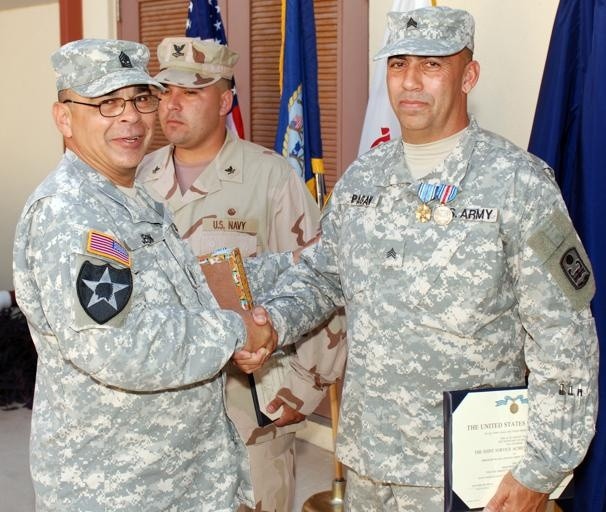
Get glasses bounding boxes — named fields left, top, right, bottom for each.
left=65, top=93, right=159, bottom=118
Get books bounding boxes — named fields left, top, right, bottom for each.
left=198, top=246, right=253, bottom=312
left=442, top=386, right=576, bottom=512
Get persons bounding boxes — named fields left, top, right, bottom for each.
left=132, top=38, right=348, bottom=512
left=231, top=5, right=599, bottom=512
left=12, top=40, right=278, bottom=512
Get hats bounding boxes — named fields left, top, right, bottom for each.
left=49, top=38, right=166, bottom=100
left=153, top=35, right=241, bottom=90
left=371, top=6, right=476, bottom=62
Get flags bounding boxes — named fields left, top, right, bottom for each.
left=274, top=1, right=328, bottom=210
left=526, top=0, right=606, bottom=512
left=183, top=1, right=245, bottom=140
left=356, top=0, right=439, bottom=157
